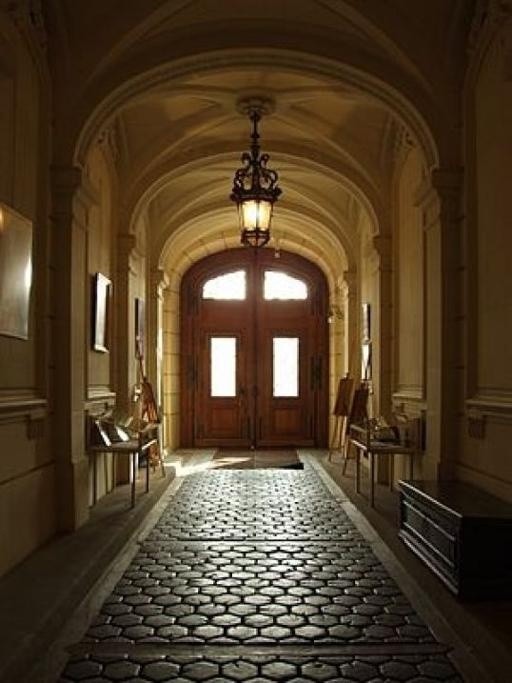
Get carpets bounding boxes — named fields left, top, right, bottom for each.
left=205, top=445, right=304, bottom=471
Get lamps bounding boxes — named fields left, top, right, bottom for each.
left=229, top=96, right=283, bottom=260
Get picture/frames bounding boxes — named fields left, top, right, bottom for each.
left=93, top=270, right=113, bottom=354
left=1, top=199, right=37, bottom=342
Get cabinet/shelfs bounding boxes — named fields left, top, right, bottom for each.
left=396, top=476, right=512, bottom=605
